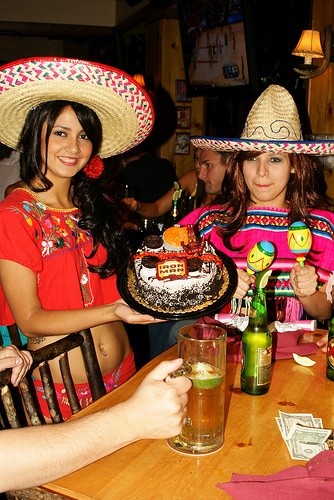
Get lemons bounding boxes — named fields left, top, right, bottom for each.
left=189, top=362, right=223, bottom=389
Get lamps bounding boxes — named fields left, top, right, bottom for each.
left=291, top=30, right=332, bottom=79
left=133, top=74, right=146, bottom=87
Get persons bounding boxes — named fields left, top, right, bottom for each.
left=123, top=146, right=216, bottom=220
left=149, top=148, right=236, bottom=356
left=0, top=344, right=192, bottom=491
left=177, top=85, right=334, bottom=329
left=0, top=57, right=167, bottom=423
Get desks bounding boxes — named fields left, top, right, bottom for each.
left=34, top=323, right=334, bottom=500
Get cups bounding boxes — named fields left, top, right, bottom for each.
left=163, top=324, right=227, bottom=455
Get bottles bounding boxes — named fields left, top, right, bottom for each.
left=168, top=199, right=179, bottom=227
left=241, top=287, right=273, bottom=395
left=326, top=318, right=334, bottom=381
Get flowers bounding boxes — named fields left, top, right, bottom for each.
left=83, top=156, right=104, bottom=179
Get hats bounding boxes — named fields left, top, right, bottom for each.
left=0, top=57, right=155, bottom=159
left=190, top=85, right=334, bottom=155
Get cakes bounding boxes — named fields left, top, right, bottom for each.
left=129, top=225, right=222, bottom=310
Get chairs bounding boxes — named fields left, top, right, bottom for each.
left=0, top=327, right=105, bottom=430
